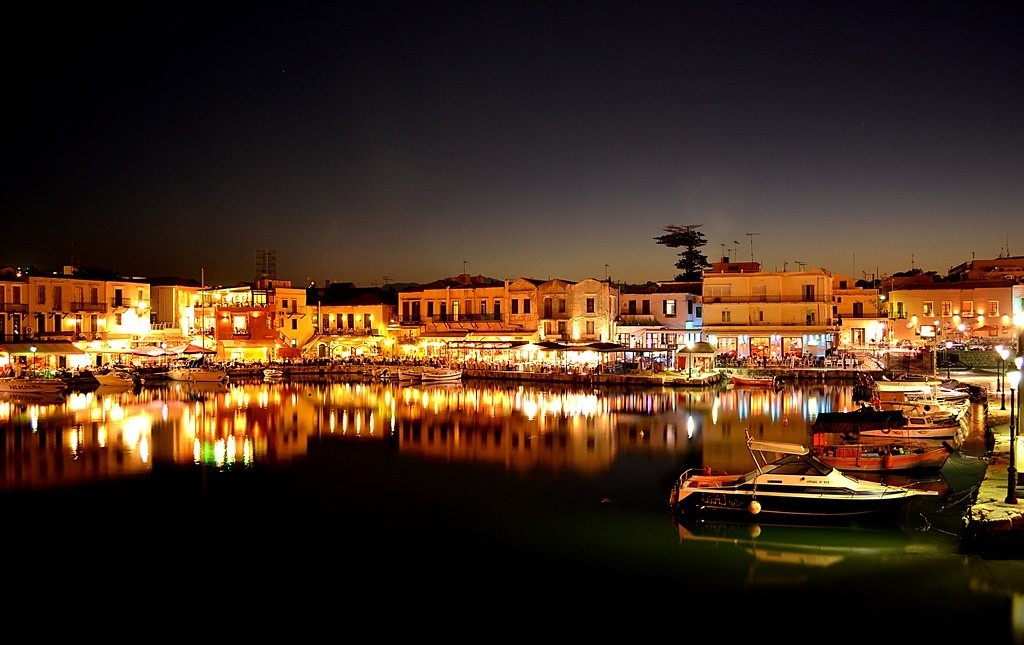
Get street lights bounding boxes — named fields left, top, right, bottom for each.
left=32, top=345, right=37, bottom=379
left=946, top=341, right=953, bottom=380
left=996, top=345, right=1003, bottom=392
left=1000, top=350, right=1007, bottom=409
left=1015, top=357, right=1024, bottom=435
left=162, top=342, right=167, bottom=366
left=1004, top=362, right=1021, bottom=504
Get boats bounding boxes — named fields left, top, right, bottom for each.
left=363, top=368, right=462, bottom=381
left=94, top=372, right=145, bottom=386
left=263, top=369, right=283, bottom=377
left=0, top=379, right=68, bottom=394
left=166, top=364, right=227, bottom=381
left=669, top=374, right=971, bottom=526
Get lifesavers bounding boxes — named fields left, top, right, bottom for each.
left=882, top=428, right=891, bottom=434
